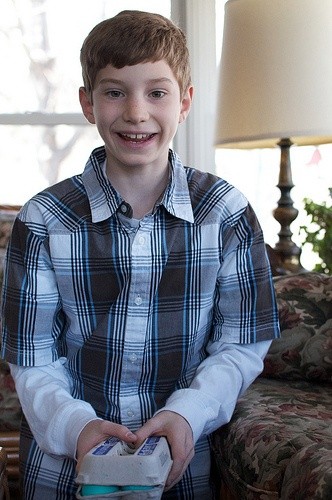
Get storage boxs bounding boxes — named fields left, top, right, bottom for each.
left=74, top=436, right=173, bottom=500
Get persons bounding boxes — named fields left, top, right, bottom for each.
left=0, top=8, right=282, bottom=500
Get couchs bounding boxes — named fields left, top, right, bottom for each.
left=0, top=204, right=332, bottom=500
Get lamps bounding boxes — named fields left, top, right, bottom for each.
left=214, top=0, right=332, bottom=275
